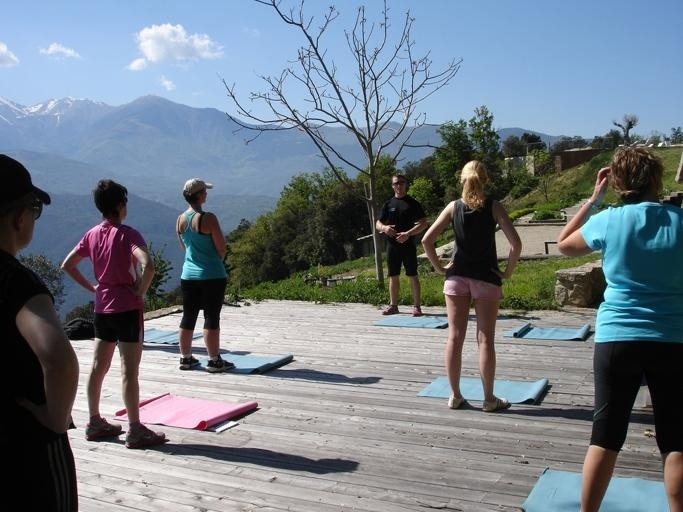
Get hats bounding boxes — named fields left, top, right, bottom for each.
left=183, top=178, right=213, bottom=196
left=0, top=154, right=51, bottom=204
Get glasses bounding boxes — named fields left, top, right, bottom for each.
left=2, top=200, right=43, bottom=220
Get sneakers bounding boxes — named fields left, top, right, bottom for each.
left=448, top=395, right=464, bottom=409
left=125, top=430, right=165, bottom=448
left=86, top=418, right=122, bottom=437
left=180, top=356, right=199, bottom=369
left=482, top=398, right=508, bottom=411
left=382, top=306, right=398, bottom=314
left=205, top=359, right=233, bottom=372
left=412, top=307, right=422, bottom=316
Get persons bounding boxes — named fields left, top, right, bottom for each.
left=176, top=178, right=235, bottom=373
left=557, top=144, right=683, bottom=511
left=421, top=160, right=522, bottom=413
left=1, top=152, right=79, bottom=512
left=376, top=172, right=427, bottom=316
left=60, top=178, right=165, bottom=448
